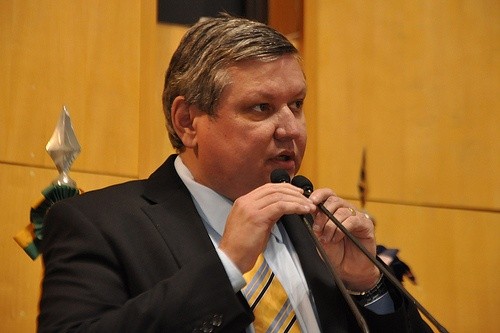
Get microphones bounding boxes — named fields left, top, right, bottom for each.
left=271, top=169, right=369, bottom=333
left=290, top=175, right=449, bottom=333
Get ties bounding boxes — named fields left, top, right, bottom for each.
left=241, top=252, right=303, bottom=333
